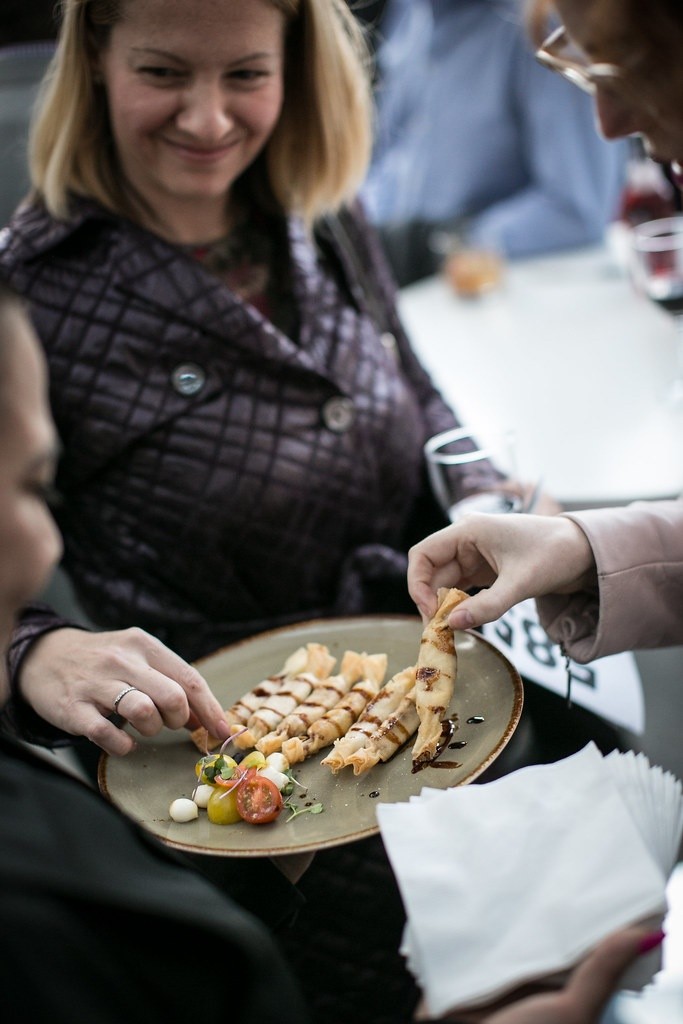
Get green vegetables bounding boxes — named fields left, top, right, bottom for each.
left=203, top=757, right=323, bottom=823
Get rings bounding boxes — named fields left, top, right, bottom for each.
left=114, top=686, right=137, bottom=717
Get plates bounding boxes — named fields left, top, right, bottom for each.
left=97, top=617, right=524, bottom=857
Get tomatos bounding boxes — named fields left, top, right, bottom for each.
left=195, top=753, right=283, bottom=824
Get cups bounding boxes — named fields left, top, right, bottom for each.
left=426, top=427, right=523, bottom=523
left=634, top=217, right=683, bottom=299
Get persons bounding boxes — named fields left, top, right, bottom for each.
left=0, top=258, right=672, bottom=1024
left=0, top=0, right=540, bottom=755
left=403, top=0, right=683, bottom=671
left=325, top=0, right=626, bottom=295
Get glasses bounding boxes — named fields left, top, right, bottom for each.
left=535, top=25, right=650, bottom=99
